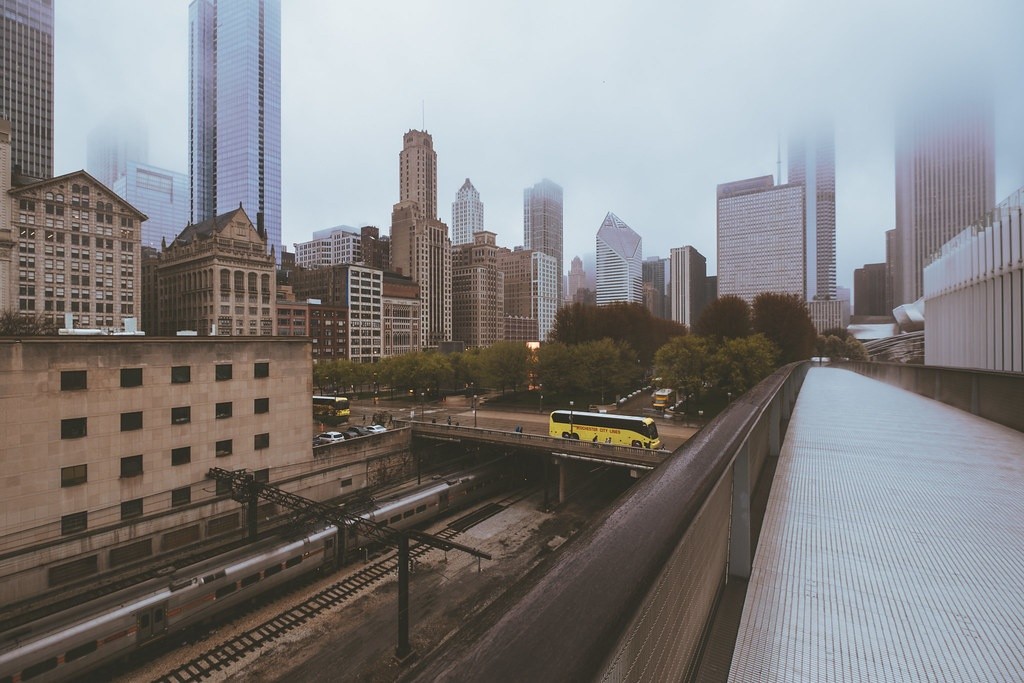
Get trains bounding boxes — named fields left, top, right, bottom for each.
left=0, top=457, right=511, bottom=683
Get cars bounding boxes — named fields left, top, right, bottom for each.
left=316, top=425, right=386, bottom=443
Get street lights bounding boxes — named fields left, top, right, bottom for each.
left=421, top=393, right=424, bottom=420
left=570, top=401, right=574, bottom=439
left=698, top=410, right=703, bottom=430
left=728, top=393, right=731, bottom=404
left=540, top=384, right=542, bottom=412
left=474, top=395, right=477, bottom=427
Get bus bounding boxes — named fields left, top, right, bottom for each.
left=313, top=396, right=351, bottom=416
left=549, top=410, right=660, bottom=450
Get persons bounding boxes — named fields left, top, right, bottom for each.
left=647, top=441, right=651, bottom=449
left=605, top=437, right=611, bottom=444
left=447, top=416, right=452, bottom=425
left=633, top=440, right=642, bottom=448
left=593, top=436, right=598, bottom=448
left=362, top=414, right=377, bottom=425
left=661, top=444, right=665, bottom=450
left=516, top=426, right=523, bottom=437
left=389, top=414, right=393, bottom=424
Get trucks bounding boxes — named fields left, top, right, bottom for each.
left=653, top=389, right=675, bottom=409
